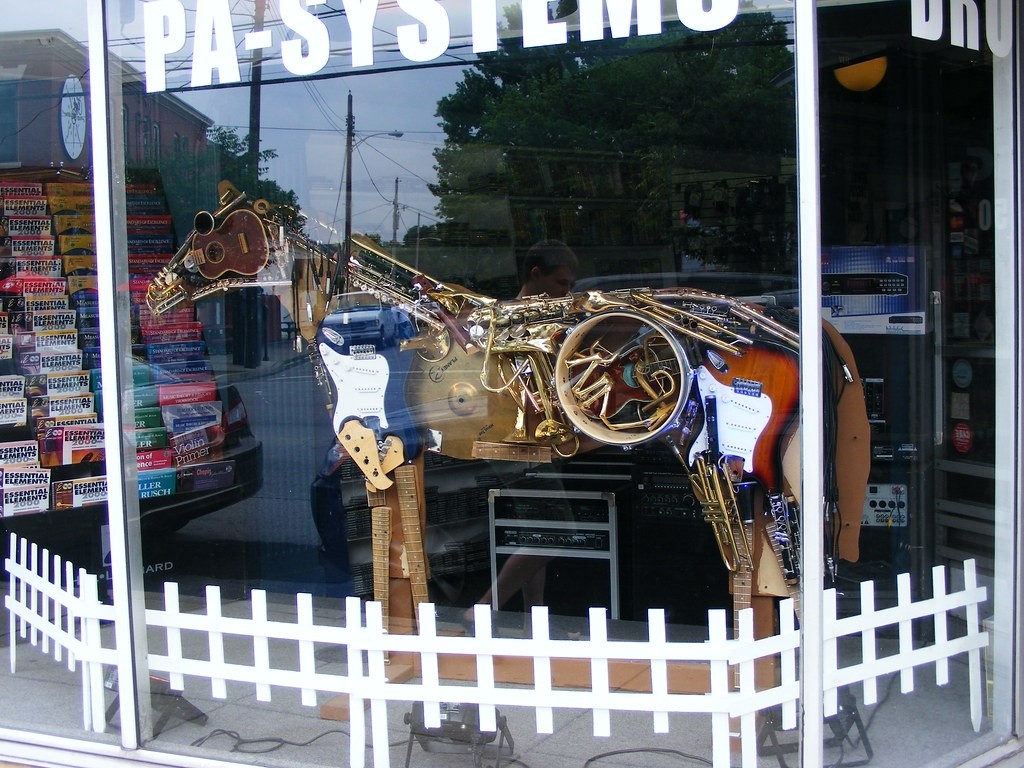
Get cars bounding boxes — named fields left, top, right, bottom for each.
left=0, top=352, right=731, bottom=620
left=317, top=289, right=401, bottom=355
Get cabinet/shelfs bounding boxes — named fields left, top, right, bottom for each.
left=315, top=141, right=993, bottom=597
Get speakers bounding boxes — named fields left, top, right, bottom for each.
left=488, top=463, right=735, bottom=628
left=841, top=333, right=911, bottom=589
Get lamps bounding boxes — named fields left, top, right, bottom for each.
left=828, top=51, right=888, bottom=92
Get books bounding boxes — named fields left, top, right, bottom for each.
left=0, top=175, right=235, bottom=519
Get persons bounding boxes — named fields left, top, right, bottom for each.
left=462, top=239, right=592, bottom=638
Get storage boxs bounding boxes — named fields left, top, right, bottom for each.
left=821, top=243, right=932, bottom=317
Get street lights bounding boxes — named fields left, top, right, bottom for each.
left=414, top=236, right=442, bottom=278
left=337, top=131, right=405, bottom=308
